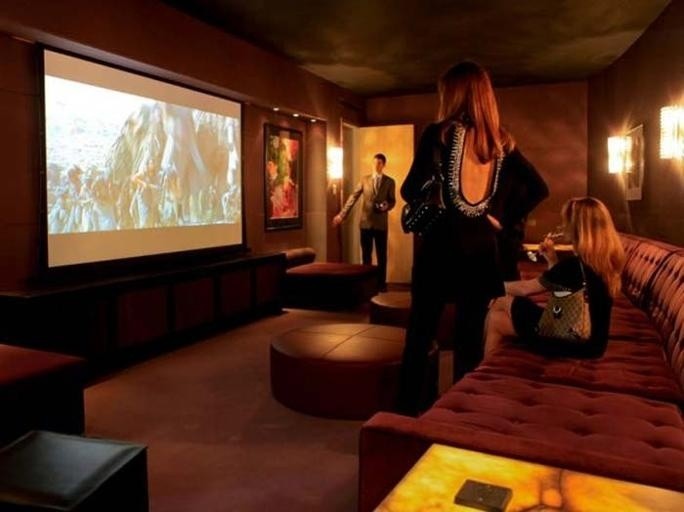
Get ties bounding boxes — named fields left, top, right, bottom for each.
left=374, top=178, right=378, bottom=193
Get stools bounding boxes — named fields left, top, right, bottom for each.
left=0, top=423, right=152, bottom=511
left=269, top=291, right=455, bottom=417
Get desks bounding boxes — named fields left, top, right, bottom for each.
left=371, top=438, right=684, bottom=511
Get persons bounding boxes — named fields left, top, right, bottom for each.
left=394, top=56, right=550, bottom=417
left=479, top=196, right=626, bottom=366
left=46, top=102, right=240, bottom=235
left=331, top=153, right=395, bottom=294
left=264, top=156, right=284, bottom=192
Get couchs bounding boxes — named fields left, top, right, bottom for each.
left=360, top=231, right=684, bottom=510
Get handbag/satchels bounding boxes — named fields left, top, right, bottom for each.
left=534, top=286, right=591, bottom=341
left=401, top=177, right=446, bottom=238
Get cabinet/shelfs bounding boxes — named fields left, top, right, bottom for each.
left=113, top=261, right=288, bottom=351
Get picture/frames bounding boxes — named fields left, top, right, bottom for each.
left=623, top=121, right=645, bottom=203
left=263, top=124, right=302, bottom=231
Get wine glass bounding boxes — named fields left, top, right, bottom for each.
left=527, top=226, right=564, bottom=262
